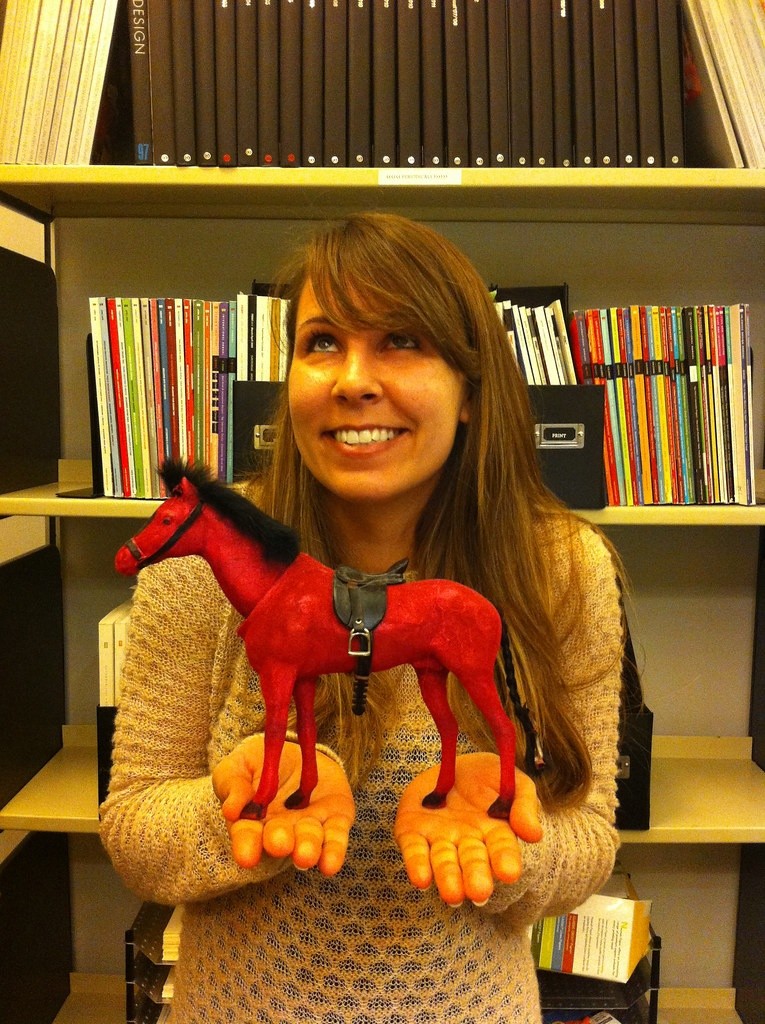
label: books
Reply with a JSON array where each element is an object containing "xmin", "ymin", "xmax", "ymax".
[
  {"xmin": 87, "ymin": 292, "xmax": 756, "ymax": 509},
  {"xmin": 94, "ymin": 597, "xmax": 135, "ymax": 709},
  {"xmin": 0, "ymin": 0, "xmax": 764, "ymax": 167},
  {"xmin": 153, "ymin": 857, "xmax": 653, "ymax": 1024}
]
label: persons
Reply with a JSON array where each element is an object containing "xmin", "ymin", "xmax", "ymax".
[{"xmin": 97, "ymin": 211, "xmax": 647, "ymax": 1024}]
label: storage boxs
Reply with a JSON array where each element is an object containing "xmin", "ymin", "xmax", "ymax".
[
  {"xmin": 230, "ymin": 377, "xmax": 605, "ymax": 512},
  {"xmin": 97, "ymin": 703, "xmax": 119, "ymax": 820}
]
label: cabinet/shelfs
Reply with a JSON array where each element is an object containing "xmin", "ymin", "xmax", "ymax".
[
  {"xmin": 526, "ymin": 863, "xmax": 663, "ymax": 1022},
  {"xmin": 0, "ymin": 162, "xmax": 765, "ymax": 1024},
  {"xmin": 124, "ymin": 894, "xmax": 183, "ymax": 1023}
]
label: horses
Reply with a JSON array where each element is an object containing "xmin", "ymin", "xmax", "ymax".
[{"xmin": 114, "ymin": 456, "xmax": 518, "ymax": 821}]
